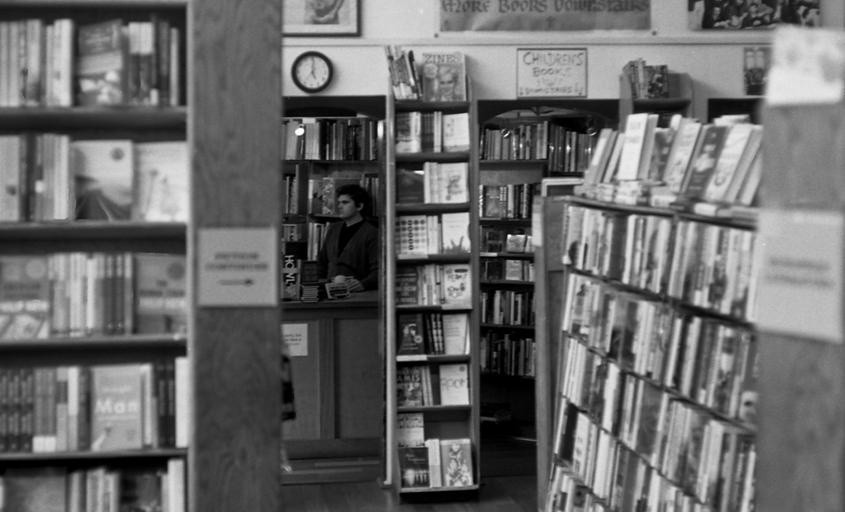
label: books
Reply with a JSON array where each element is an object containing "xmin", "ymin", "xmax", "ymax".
[
  {"xmin": 384, "ymin": 44, "xmax": 770, "ymax": 512},
  {"xmin": 1, "ymin": 13, "xmax": 189, "ymax": 510},
  {"xmin": 282, "ymin": 117, "xmax": 384, "ymax": 218},
  {"xmin": 282, "ymin": 223, "xmax": 329, "ymax": 302}
]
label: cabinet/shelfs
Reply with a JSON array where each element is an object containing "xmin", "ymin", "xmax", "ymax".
[
  {"xmin": 707, "ymin": 96, "xmax": 764, "ymax": 124},
  {"xmin": 384, "ymin": 75, "xmax": 478, "ymax": 499},
  {"xmin": 528, "ymin": 194, "xmax": 765, "ymax": 511},
  {"xmin": 0, "ymin": 0, "xmax": 282, "ymax": 510},
  {"xmin": 282, "ymin": 116, "xmax": 384, "ymax": 288},
  {"xmin": 477, "ymin": 97, "xmax": 692, "ymax": 414}
]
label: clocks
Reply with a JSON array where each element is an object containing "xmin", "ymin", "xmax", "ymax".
[{"xmin": 291, "ymin": 51, "xmax": 332, "ymax": 92}]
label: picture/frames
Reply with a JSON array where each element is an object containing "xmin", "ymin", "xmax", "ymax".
[
  {"xmin": 282, "ymin": 0, "xmax": 361, "ymax": 37},
  {"xmin": 515, "ymin": 47, "xmax": 588, "ymax": 98}
]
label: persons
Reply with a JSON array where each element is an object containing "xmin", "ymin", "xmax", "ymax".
[
  {"xmin": 688, "ymin": 0, "xmax": 823, "ymax": 34},
  {"xmin": 304, "ymin": 1, "xmax": 344, "ymax": 25},
  {"xmin": 316, "ymin": 184, "xmax": 378, "ymax": 294}
]
